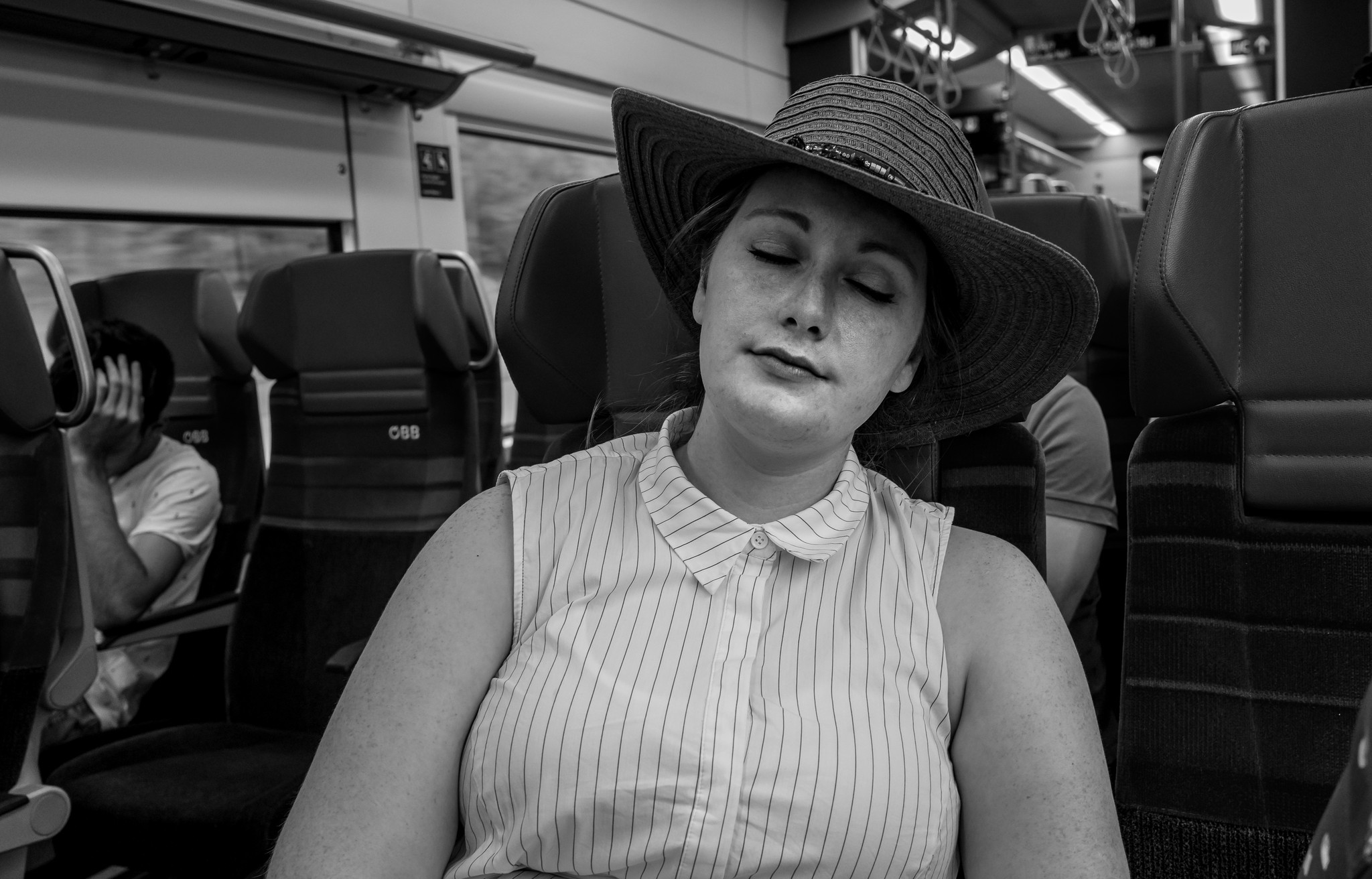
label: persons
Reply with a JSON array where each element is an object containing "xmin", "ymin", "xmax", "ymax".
[
  {"xmin": 35, "ymin": 321, "xmax": 221, "ymax": 767},
  {"xmin": 269, "ymin": 75, "xmax": 1130, "ymax": 879},
  {"xmin": 1017, "ymin": 375, "xmax": 1117, "ymax": 628}
]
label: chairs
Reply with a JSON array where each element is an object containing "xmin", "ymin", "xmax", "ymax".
[{"xmin": 0, "ymin": 85, "xmax": 1371, "ymax": 879}]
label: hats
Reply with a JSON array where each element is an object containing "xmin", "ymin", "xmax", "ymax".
[{"xmin": 608, "ymin": 75, "xmax": 1101, "ymax": 450}]
